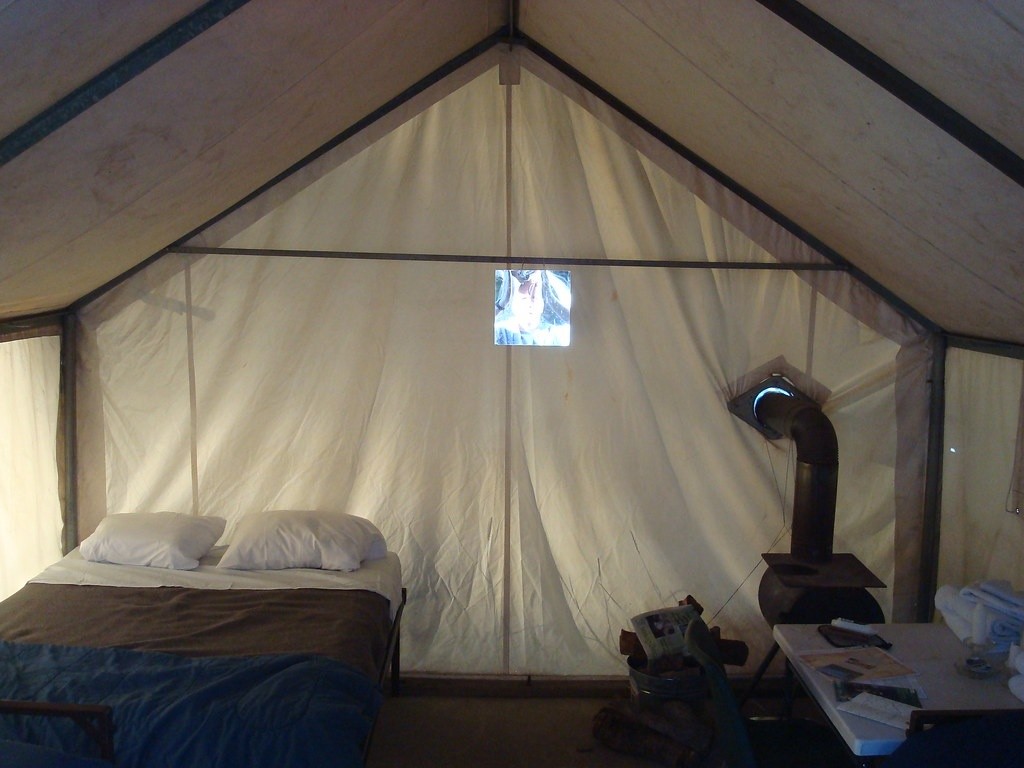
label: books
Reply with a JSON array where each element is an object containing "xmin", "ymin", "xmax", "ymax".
[{"xmin": 803, "ymin": 644, "xmax": 932, "ymax": 733}]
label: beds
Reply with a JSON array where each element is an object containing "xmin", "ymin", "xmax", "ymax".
[{"xmin": 1, "ymin": 544, "xmax": 407, "ymax": 768}]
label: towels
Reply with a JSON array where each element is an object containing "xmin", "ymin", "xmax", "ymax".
[{"xmin": 934, "ymin": 576, "xmax": 1024, "ymax": 655}]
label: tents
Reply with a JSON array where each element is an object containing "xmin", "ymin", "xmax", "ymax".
[{"xmin": 0, "ymin": 0, "xmax": 1024, "ymax": 695}]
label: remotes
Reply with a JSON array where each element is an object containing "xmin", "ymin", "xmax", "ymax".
[{"xmin": 831, "ymin": 620, "xmax": 874, "ymax": 636}]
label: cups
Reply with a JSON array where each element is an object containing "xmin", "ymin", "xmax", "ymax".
[{"xmin": 961, "ymin": 637, "xmax": 995, "ymax": 678}]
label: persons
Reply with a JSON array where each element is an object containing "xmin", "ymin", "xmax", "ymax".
[{"xmin": 495, "ymin": 280, "xmax": 570, "ymax": 346}]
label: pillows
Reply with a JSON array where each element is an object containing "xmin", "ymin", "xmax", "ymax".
[
  {"xmin": 78, "ymin": 511, "xmax": 226, "ymax": 570},
  {"xmin": 218, "ymin": 509, "xmax": 389, "ymax": 572}
]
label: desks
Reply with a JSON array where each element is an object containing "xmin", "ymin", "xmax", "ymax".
[{"xmin": 772, "ymin": 622, "xmax": 1024, "ymax": 757}]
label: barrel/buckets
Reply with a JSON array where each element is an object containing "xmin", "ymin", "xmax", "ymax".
[{"xmin": 627, "ymin": 657, "xmax": 709, "ymax": 718}]
